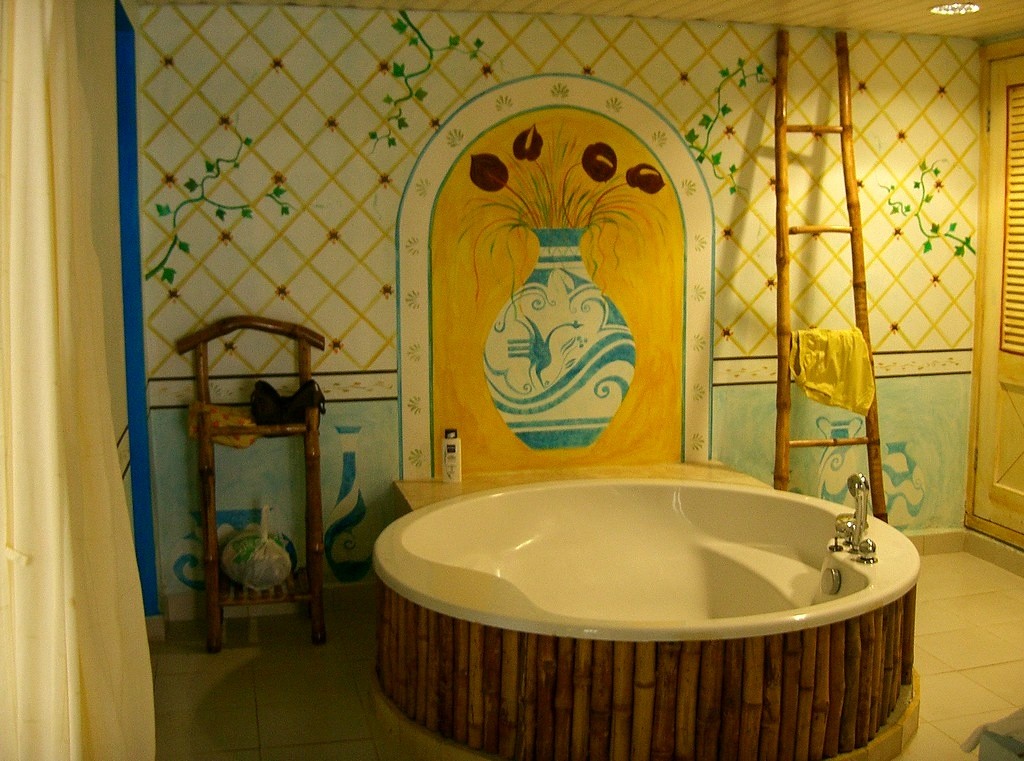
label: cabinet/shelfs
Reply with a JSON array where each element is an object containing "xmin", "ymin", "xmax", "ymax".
[{"xmin": 176, "ymin": 314, "xmax": 327, "ymax": 650}]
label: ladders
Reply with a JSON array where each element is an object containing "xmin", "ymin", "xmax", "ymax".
[{"xmin": 772, "ymin": 29, "xmax": 891, "ymax": 527}]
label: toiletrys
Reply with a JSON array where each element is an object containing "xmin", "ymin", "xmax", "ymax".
[{"xmin": 441, "ymin": 428, "xmax": 462, "ymax": 483}]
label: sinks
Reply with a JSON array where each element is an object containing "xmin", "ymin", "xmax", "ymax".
[{"xmin": 373, "ymin": 478, "xmax": 920, "ymax": 761}]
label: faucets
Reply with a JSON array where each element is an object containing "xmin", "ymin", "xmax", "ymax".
[{"xmin": 847, "ymin": 472, "xmax": 869, "ymax": 554}]
